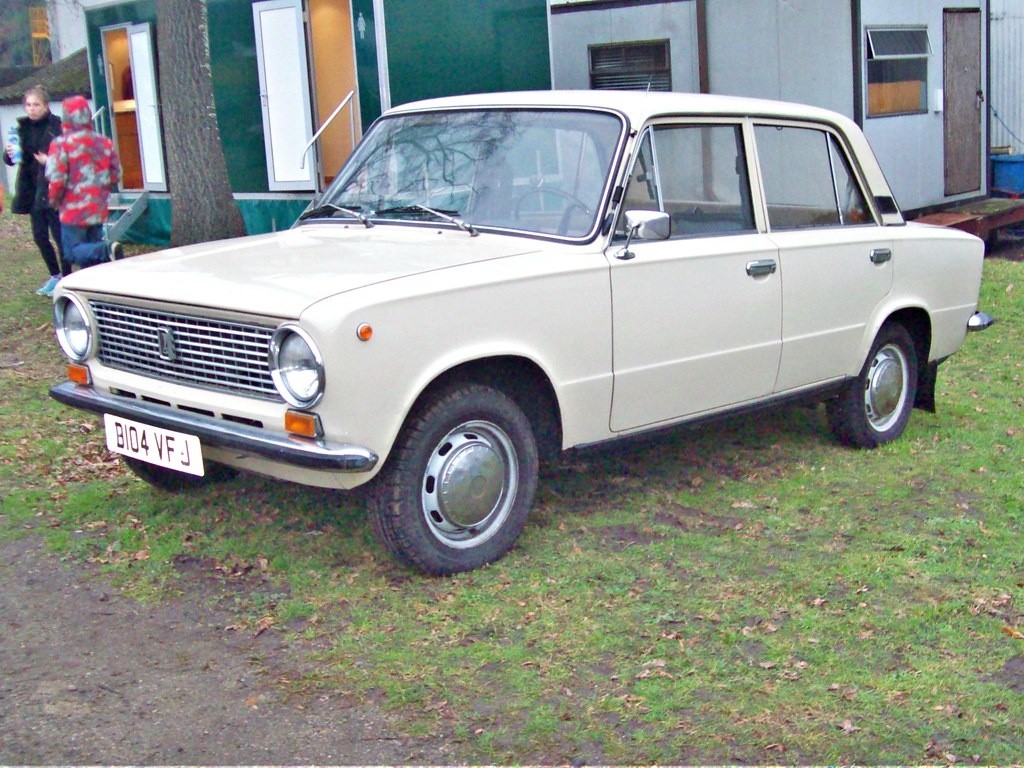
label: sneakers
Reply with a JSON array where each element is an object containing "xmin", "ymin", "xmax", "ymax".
[
  {"xmin": 36, "ymin": 274, "xmax": 61, "ymax": 298},
  {"xmin": 111, "ymin": 242, "xmax": 125, "ymax": 260}
]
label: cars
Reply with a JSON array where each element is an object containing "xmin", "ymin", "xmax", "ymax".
[{"xmin": 47, "ymin": 88, "xmax": 985, "ymax": 578}]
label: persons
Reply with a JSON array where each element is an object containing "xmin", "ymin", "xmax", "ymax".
[
  {"xmin": 45, "ymin": 96, "xmax": 123, "ymax": 269},
  {"xmin": 3, "ymin": 84, "xmax": 73, "ymax": 297}
]
